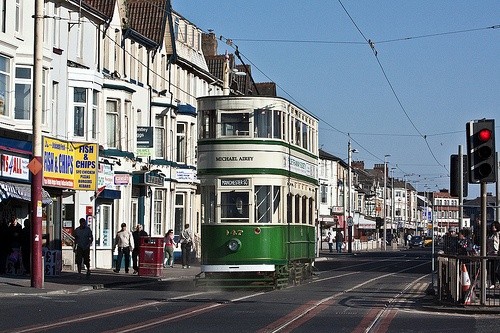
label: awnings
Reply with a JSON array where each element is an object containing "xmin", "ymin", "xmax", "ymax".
[
  {"xmin": 0, "ymin": 180, "xmax": 52, "ymax": 203},
  {"xmin": 98, "ymin": 189, "xmax": 120, "ymax": 199}
]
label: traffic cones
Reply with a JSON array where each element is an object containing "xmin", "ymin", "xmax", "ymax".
[{"xmin": 461, "ymin": 263, "xmax": 475, "ymax": 305}]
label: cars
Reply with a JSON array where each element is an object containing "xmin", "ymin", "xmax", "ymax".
[{"xmin": 409, "ymin": 235, "xmax": 438, "ymax": 249}]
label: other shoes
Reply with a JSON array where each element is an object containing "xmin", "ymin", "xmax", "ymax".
[
  {"xmin": 125, "ymin": 269, "xmax": 129, "ymax": 273},
  {"xmin": 182, "ymin": 266, "xmax": 190, "ymax": 269},
  {"xmin": 133, "ymin": 270, "xmax": 138, "ymax": 274},
  {"xmin": 169, "ymin": 266, "xmax": 173, "ymax": 268},
  {"xmin": 113, "ymin": 269, "xmax": 119, "ymax": 273},
  {"xmin": 163, "ymin": 264, "xmax": 166, "ymax": 268},
  {"xmin": 86, "ymin": 270, "xmax": 91, "ymax": 277},
  {"xmin": 490, "ymin": 285, "xmax": 495, "ymax": 289},
  {"xmin": 76, "ymin": 274, "xmax": 83, "ymax": 279}
]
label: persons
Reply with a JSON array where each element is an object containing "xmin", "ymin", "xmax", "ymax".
[
  {"xmin": 176, "ymin": 224, "xmax": 194, "ymax": 269},
  {"xmin": 328, "ymin": 232, "xmax": 335, "ymax": 252},
  {"xmin": 73, "ymin": 218, "xmax": 93, "ymax": 280},
  {"xmin": 444, "ymin": 226, "xmax": 472, "ymax": 281},
  {"xmin": 112, "ymin": 223, "xmax": 135, "ymax": 273},
  {"xmin": 486, "ymin": 221, "xmax": 500, "ymax": 289},
  {"xmin": 0, "ymin": 219, "xmax": 30, "ymax": 278},
  {"xmin": 404, "ymin": 233, "xmax": 412, "ymax": 246},
  {"xmin": 163, "ymin": 229, "xmax": 178, "ymax": 268},
  {"xmin": 335, "ymin": 231, "xmax": 343, "ymax": 254},
  {"xmin": 132, "ymin": 224, "xmax": 149, "ymax": 274}
]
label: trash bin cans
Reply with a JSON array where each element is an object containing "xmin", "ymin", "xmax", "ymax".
[
  {"xmin": 138, "ymin": 237, "xmax": 165, "ymax": 276},
  {"xmin": 393, "ymin": 241, "xmax": 397, "ymax": 249},
  {"xmin": 381, "ymin": 241, "xmax": 384, "ymax": 250}
]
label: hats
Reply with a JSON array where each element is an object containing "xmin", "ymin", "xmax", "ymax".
[
  {"xmin": 121, "ymin": 223, "xmax": 126, "ymax": 227},
  {"xmin": 448, "ymin": 227, "xmax": 455, "ymax": 232}
]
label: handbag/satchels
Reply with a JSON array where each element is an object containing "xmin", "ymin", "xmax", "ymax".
[{"xmin": 467, "ymin": 247, "xmax": 478, "ymax": 262}]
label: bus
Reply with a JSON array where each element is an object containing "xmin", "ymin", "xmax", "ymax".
[{"xmin": 195, "ymin": 51, "xmax": 322, "ymax": 291}]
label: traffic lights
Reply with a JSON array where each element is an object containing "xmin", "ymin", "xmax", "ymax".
[{"xmin": 466, "ymin": 118, "xmax": 497, "ymax": 183}]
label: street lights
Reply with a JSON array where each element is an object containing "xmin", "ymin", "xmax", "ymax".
[
  {"xmin": 390, "ymin": 167, "xmax": 397, "ymax": 250},
  {"xmin": 347, "ymin": 139, "xmax": 359, "ymax": 254},
  {"xmin": 404, "ymin": 181, "xmax": 410, "ymax": 229},
  {"xmin": 383, "ymin": 154, "xmax": 391, "ymax": 250},
  {"xmin": 422, "ymin": 186, "xmax": 431, "ymax": 236}
]
